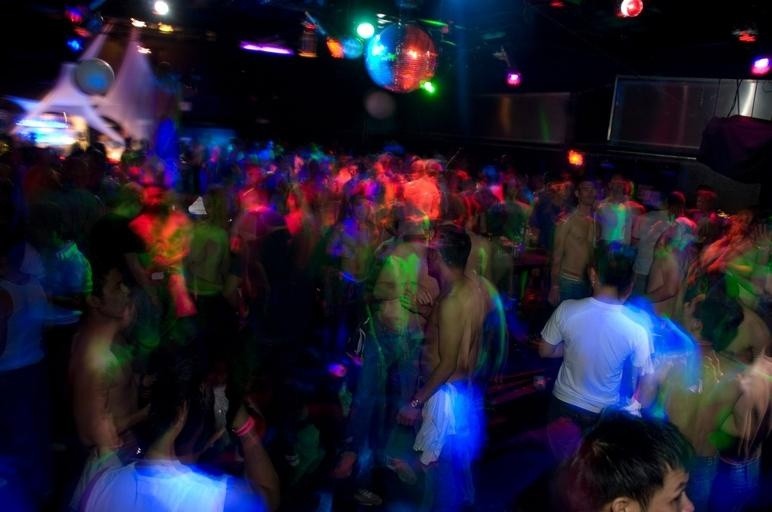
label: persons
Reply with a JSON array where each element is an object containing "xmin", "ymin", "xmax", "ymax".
[{"xmin": 1, "ymin": 126, "xmax": 772, "ymax": 512}]
